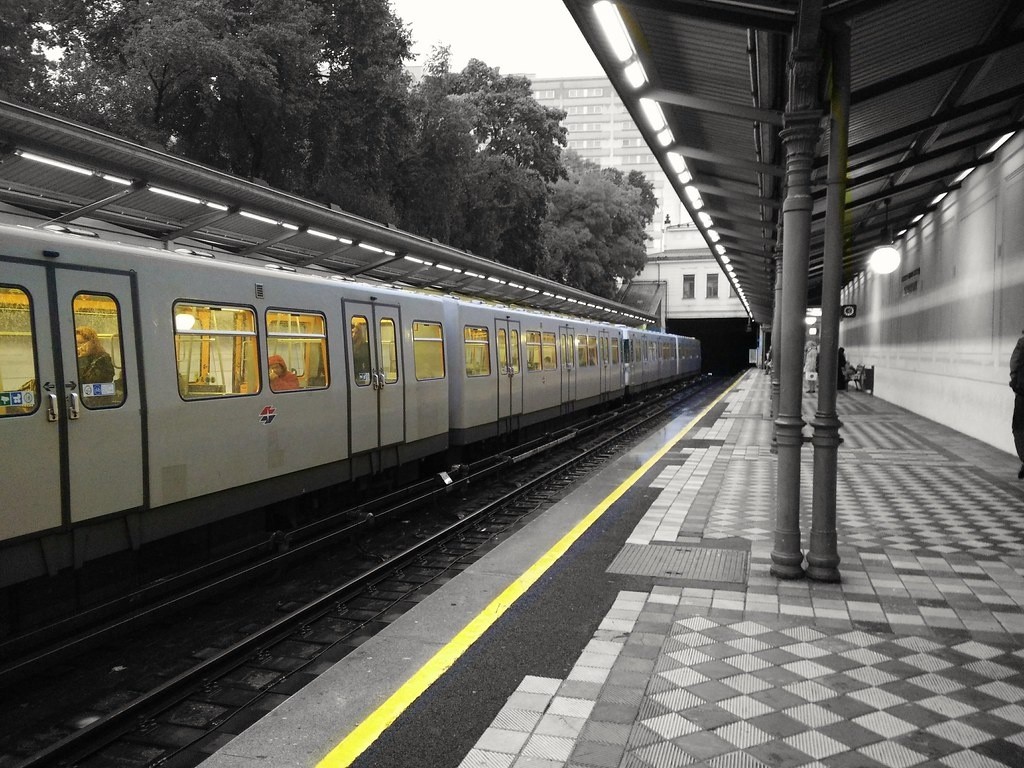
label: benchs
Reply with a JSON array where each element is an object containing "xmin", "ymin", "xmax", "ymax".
[
  {"xmin": 845, "ymin": 364, "xmax": 865, "ymax": 392},
  {"xmin": 184, "ymin": 384, "xmax": 224, "ymax": 397}
]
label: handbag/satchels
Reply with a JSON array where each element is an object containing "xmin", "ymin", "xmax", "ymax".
[{"xmin": 806, "ymin": 372, "xmax": 818, "ymax": 380}]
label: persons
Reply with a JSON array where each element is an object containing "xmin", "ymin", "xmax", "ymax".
[
  {"xmin": 765, "ymin": 346, "xmax": 773, "ymax": 375},
  {"xmin": 351, "ymin": 323, "xmax": 370, "ymax": 386},
  {"xmin": 1008, "ymin": 328, "xmax": 1024, "ymax": 479},
  {"xmin": 804, "ymin": 341, "xmax": 865, "ymax": 393},
  {"xmin": 269, "ymin": 355, "xmax": 300, "ymax": 392},
  {"xmin": 75, "ymin": 326, "xmax": 114, "ymax": 407}
]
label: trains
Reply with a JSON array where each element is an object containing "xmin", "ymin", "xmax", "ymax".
[{"xmin": 1, "ymin": 222, "xmax": 702, "ymax": 591}]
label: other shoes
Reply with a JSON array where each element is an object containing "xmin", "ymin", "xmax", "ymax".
[
  {"xmin": 806, "ymin": 390, "xmax": 811, "ymax": 393},
  {"xmin": 763, "ymin": 373, "xmax": 768, "ymax": 375},
  {"xmin": 810, "ymin": 391, "xmax": 815, "ymax": 393}
]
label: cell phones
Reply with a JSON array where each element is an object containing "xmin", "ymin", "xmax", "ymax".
[{"xmin": 78, "ymin": 340, "xmax": 94, "ymax": 354}]
